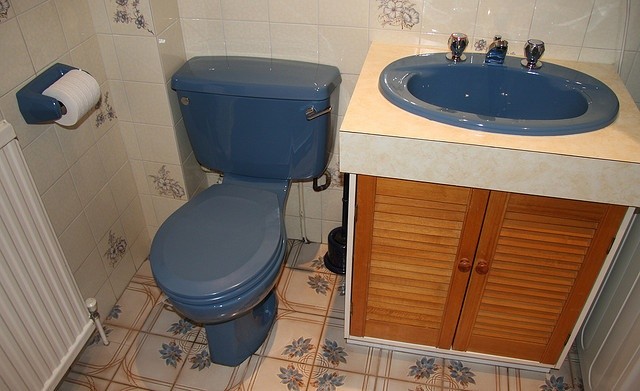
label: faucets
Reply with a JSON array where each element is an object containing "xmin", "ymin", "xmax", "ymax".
[{"xmin": 485, "ymin": 40, "xmax": 508, "ymax": 62}]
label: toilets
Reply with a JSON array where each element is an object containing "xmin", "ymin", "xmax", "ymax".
[{"xmin": 150, "ymin": 57, "xmax": 342, "ymax": 366}]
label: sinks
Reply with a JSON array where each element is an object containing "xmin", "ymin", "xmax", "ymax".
[{"xmin": 408, "ymin": 64, "xmax": 589, "ymax": 120}]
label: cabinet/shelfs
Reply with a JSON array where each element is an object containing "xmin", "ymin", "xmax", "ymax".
[{"xmin": 349, "ymin": 174, "xmax": 630, "ymax": 368}]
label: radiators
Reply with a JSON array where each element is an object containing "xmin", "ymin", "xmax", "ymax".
[{"xmin": 0, "ymin": 120, "xmax": 110, "ymax": 390}]
